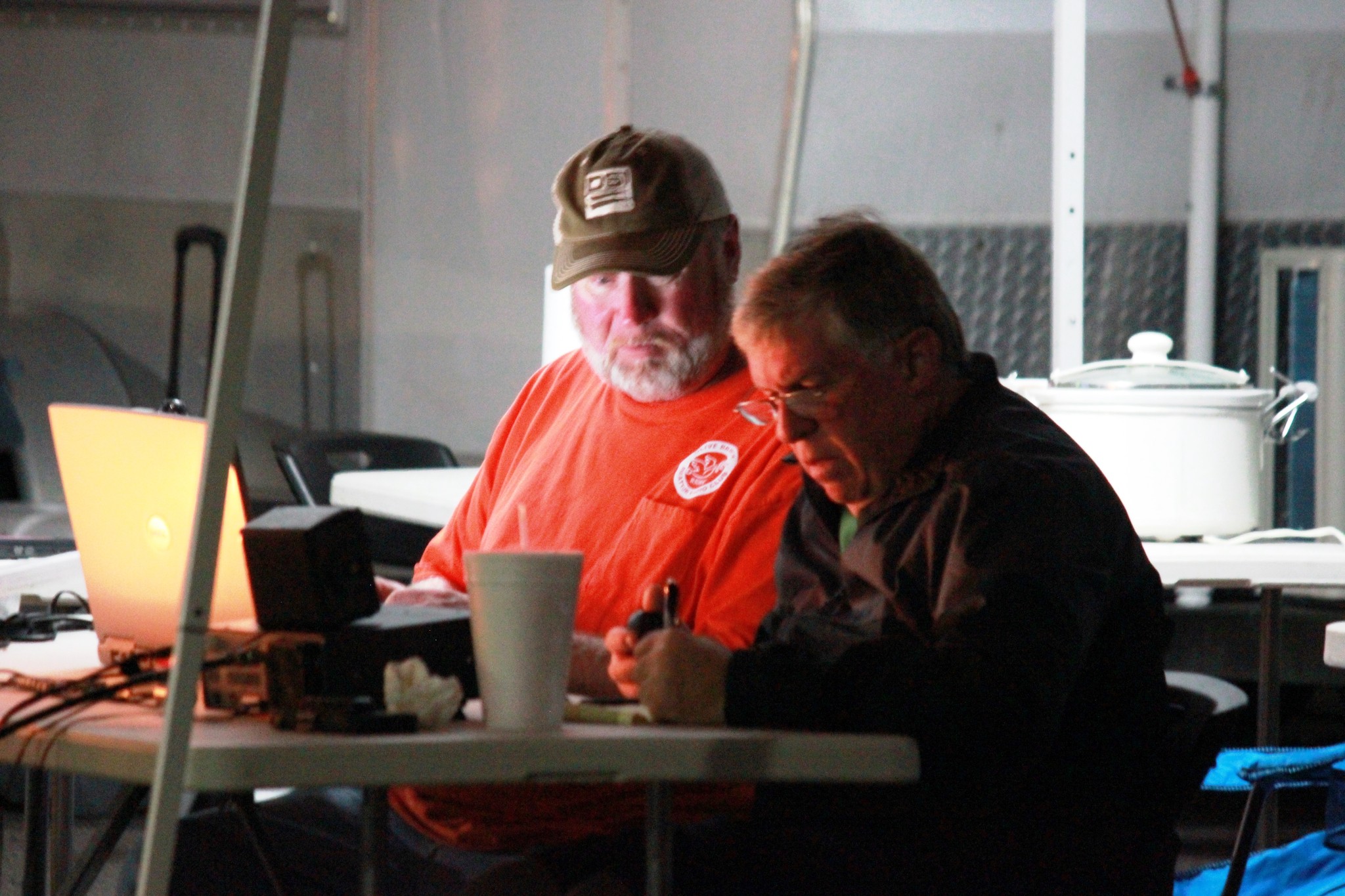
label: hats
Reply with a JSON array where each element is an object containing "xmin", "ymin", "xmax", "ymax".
[{"xmin": 550, "ymin": 127, "xmax": 730, "ymax": 292}]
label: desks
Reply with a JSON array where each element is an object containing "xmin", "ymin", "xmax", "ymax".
[
  {"xmin": 1142, "ymin": 543, "xmax": 1345, "ymax": 841},
  {"xmin": 0, "ymin": 680, "xmax": 918, "ymax": 896}
]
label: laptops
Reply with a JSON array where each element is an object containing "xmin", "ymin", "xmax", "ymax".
[{"xmin": 46, "ymin": 404, "xmax": 262, "ymax": 662}]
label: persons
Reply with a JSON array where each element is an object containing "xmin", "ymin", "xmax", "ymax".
[
  {"xmin": 606, "ymin": 213, "xmax": 1188, "ymax": 896},
  {"xmin": 116, "ymin": 123, "xmax": 807, "ymax": 896}
]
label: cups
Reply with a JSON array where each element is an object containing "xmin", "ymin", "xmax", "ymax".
[{"xmin": 461, "ymin": 548, "xmax": 584, "ymax": 733}]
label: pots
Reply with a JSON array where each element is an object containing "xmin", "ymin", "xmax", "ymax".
[{"xmin": 1037, "ymin": 331, "xmax": 1276, "ymax": 540}]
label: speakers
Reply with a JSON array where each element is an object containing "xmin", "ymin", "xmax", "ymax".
[{"xmin": 242, "ymin": 507, "xmax": 379, "ymax": 632}]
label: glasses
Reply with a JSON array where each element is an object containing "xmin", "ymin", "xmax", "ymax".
[{"xmin": 734, "ymin": 329, "xmax": 921, "ymax": 428}]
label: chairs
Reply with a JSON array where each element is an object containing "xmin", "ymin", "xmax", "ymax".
[{"xmin": 271, "ymin": 432, "xmax": 457, "ymax": 507}]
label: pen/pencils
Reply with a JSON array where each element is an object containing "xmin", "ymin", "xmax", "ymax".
[{"xmin": 662, "ymin": 577, "xmax": 679, "ymax": 627}]
